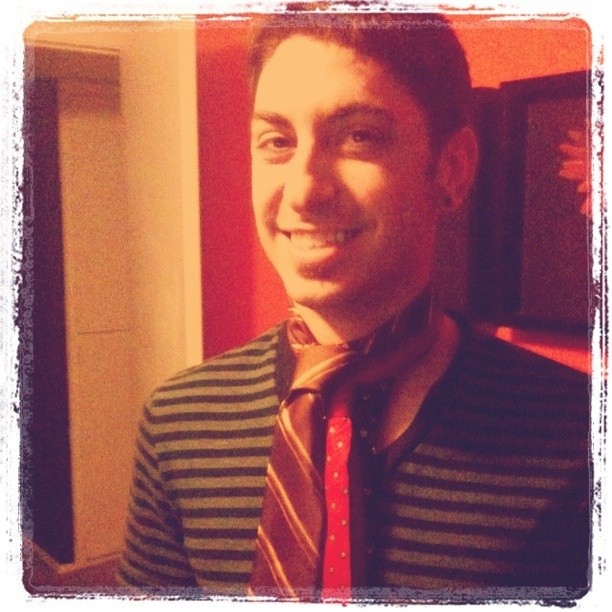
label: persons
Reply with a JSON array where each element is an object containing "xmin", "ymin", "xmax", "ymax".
[{"xmin": 113, "ymin": 13, "xmax": 591, "ymax": 587}]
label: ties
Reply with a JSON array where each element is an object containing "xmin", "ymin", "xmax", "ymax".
[{"xmin": 247, "ymin": 282, "xmax": 443, "ymax": 601}]
